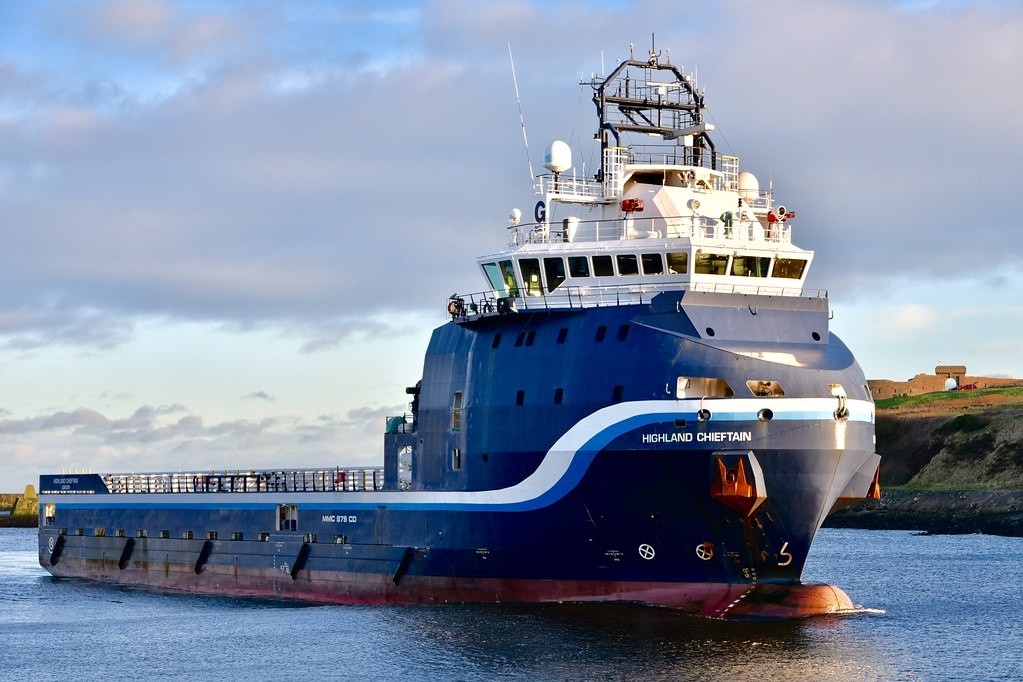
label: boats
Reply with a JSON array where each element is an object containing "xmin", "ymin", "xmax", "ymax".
[{"xmin": 39, "ymin": 36, "xmax": 880, "ymax": 622}]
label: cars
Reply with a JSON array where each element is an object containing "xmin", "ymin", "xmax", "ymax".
[{"xmin": 952, "ymin": 383, "xmax": 977, "ymax": 390}]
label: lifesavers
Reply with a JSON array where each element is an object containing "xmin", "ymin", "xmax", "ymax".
[{"xmin": 193, "ymin": 476, "xmax": 199, "ymax": 488}]
